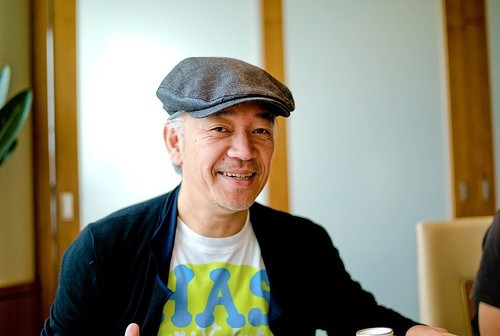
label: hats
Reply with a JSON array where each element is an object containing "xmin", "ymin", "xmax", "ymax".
[{"xmin": 156, "ymin": 57, "xmax": 295, "ymax": 119}]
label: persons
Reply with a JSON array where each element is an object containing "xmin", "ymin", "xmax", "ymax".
[
  {"xmin": 41, "ymin": 58, "xmax": 459, "ymax": 336},
  {"xmin": 469, "ymin": 207, "xmax": 500, "ymax": 336}
]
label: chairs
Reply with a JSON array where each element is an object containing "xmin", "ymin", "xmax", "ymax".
[{"xmin": 416, "ymin": 215, "xmax": 494, "ymax": 336}]
